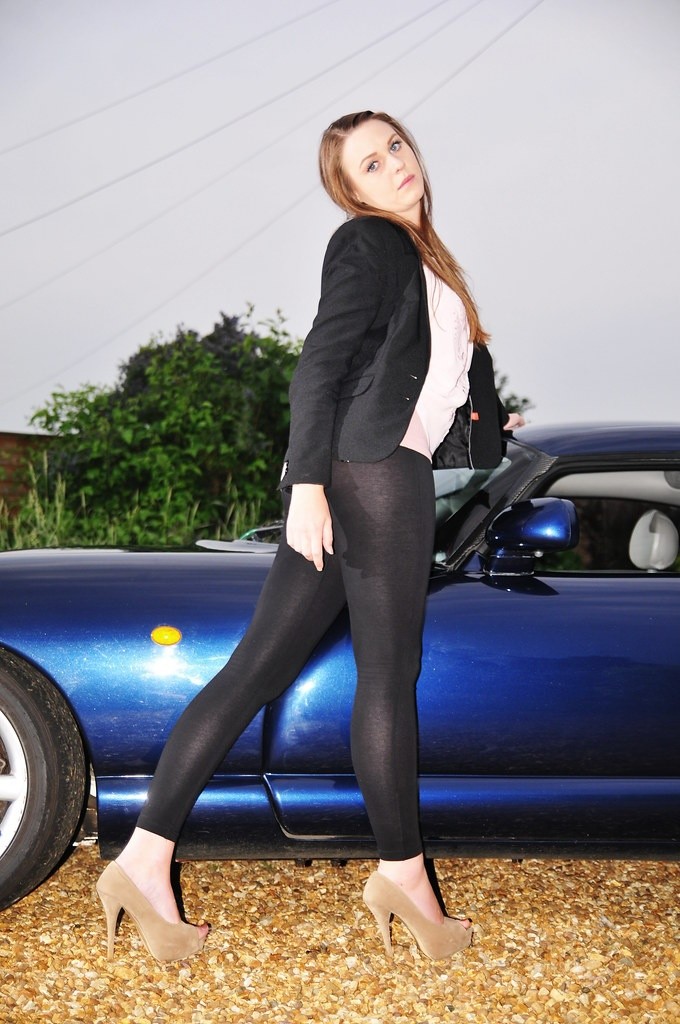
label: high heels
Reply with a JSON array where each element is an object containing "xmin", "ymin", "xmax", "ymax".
[
  {"xmin": 94, "ymin": 861, "xmax": 205, "ymax": 963},
  {"xmin": 363, "ymin": 872, "xmax": 473, "ymax": 959}
]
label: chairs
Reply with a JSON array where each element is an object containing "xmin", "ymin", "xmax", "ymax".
[{"xmin": 629, "ymin": 509, "xmax": 680, "ymax": 573}]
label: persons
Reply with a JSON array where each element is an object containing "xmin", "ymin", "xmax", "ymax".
[{"xmin": 99, "ymin": 110, "xmax": 531, "ymax": 961}]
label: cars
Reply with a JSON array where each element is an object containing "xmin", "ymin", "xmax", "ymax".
[{"xmin": 0, "ymin": 418, "xmax": 678, "ymax": 911}]
